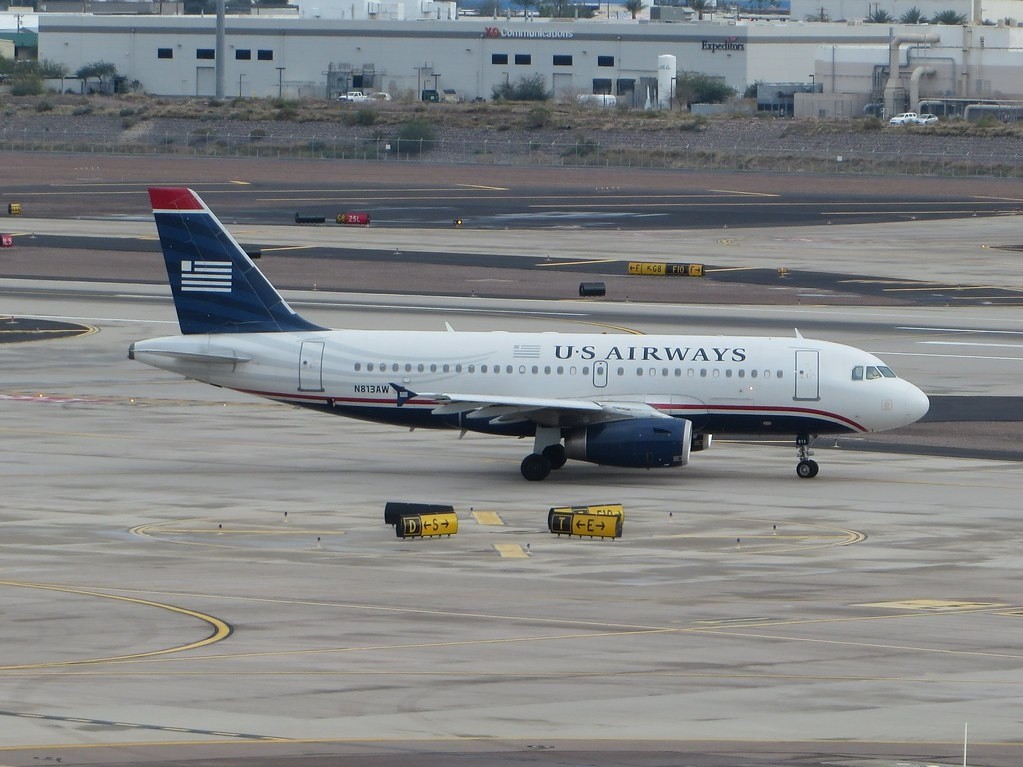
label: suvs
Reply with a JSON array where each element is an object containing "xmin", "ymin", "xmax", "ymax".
[{"xmin": 890, "ymin": 112, "xmax": 917, "ymax": 126}]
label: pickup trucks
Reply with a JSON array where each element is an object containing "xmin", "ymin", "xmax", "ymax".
[{"xmin": 336, "ymin": 91, "xmax": 367, "ymax": 103}]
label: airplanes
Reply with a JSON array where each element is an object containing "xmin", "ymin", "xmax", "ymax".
[{"xmin": 124, "ymin": 186, "xmax": 932, "ymax": 484}]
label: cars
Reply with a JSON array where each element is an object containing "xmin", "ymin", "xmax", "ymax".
[
  {"xmin": 367, "ymin": 92, "xmax": 393, "ymax": 104},
  {"xmin": 914, "ymin": 113, "xmax": 939, "ymax": 125}
]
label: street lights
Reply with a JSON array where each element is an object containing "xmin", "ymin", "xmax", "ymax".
[
  {"xmin": 670, "ymin": 77, "xmax": 677, "ymax": 111},
  {"xmin": 502, "ymin": 72, "xmax": 509, "ymax": 97},
  {"xmin": 430, "ymin": 73, "xmax": 442, "ymax": 90},
  {"xmin": 276, "ymin": 67, "xmax": 286, "ymax": 98},
  {"xmin": 239, "ymin": 73, "xmax": 247, "ymax": 97},
  {"xmin": 809, "ymin": 74, "xmax": 815, "ymax": 92}
]
